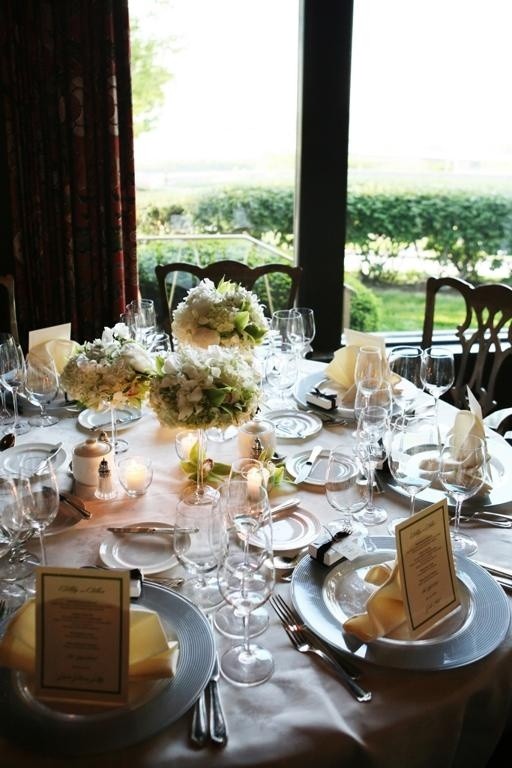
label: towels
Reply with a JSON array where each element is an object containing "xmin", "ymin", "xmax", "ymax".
[
  {"xmin": 1, "ymin": 597, "xmax": 180, "ymax": 681},
  {"xmin": 422, "ymin": 380, "xmax": 492, "ymax": 498},
  {"xmin": 13, "ymin": 335, "xmax": 81, "ymax": 388},
  {"xmin": 324, "ymin": 328, "xmax": 403, "ymax": 404},
  {"xmin": 342, "ymin": 554, "xmax": 456, "ymax": 643}
]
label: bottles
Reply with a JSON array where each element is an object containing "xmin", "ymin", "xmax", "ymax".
[{"xmin": 94, "ymin": 457, "xmax": 115, "ymax": 501}]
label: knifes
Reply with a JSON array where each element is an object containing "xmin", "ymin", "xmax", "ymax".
[
  {"xmin": 43, "ymin": 484, "xmax": 93, "ymax": 521},
  {"xmin": 293, "ymin": 444, "xmax": 326, "ymax": 487},
  {"xmin": 89, "ymin": 414, "xmax": 141, "ymax": 432},
  {"xmin": 105, "ymin": 524, "xmax": 201, "ymax": 537},
  {"xmin": 190, "ymin": 648, "xmax": 232, "ymax": 751}
]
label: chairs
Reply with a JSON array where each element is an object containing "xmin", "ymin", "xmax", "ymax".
[
  {"xmin": 415, "ymin": 276, "xmax": 512, "ymax": 447},
  {"xmin": 155, "ymin": 261, "xmax": 304, "ymax": 352}
]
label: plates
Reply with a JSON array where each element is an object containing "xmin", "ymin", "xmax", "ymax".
[
  {"xmin": 2, "ymin": 490, "xmax": 87, "ymax": 538},
  {"xmin": 1, "ymin": 442, "xmax": 68, "ymax": 474},
  {"xmin": 1, "ymin": 581, "xmax": 215, "ymax": 754},
  {"xmin": 286, "ymin": 449, "xmax": 358, "ymax": 487},
  {"xmin": 288, "ymin": 532, "xmax": 512, "ymax": 673},
  {"xmin": 296, "ymin": 368, "xmax": 368, "ymax": 416},
  {"xmin": 22, "ymin": 394, "xmax": 78, "ymax": 409},
  {"xmin": 391, "ymin": 419, "xmax": 511, "ymax": 509},
  {"xmin": 255, "ymin": 525, "xmax": 334, "ymax": 570},
  {"xmin": 94, "ymin": 520, "xmax": 193, "ymax": 577},
  {"xmin": 263, "ymin": 407, "xmax": 325, "ymax": 440},
  {"xmin": 238, "ymin": 503, "xmax": 323, "ymax": 552},
  {"xmin": 76, "ymin": 401, "xmax": 143, "ymax": 431}
]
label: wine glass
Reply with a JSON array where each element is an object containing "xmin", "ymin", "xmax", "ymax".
[
  {"xmin": 387, "ymin": 412, "xmax": 441, "ymax": 534},
  {"xmin": 350, "ymin": 344, "xmax": 392, "ymax": 526},
  {"xmin": 388, "ymin": 344, "xmax": 459, "ymax": 419},
  {"xmin": 119, "ymin": 298, "xmax": 172, "ymax": 354},
  {"xmin": 172, "ymin": 494, "xmax": 223, "ymax": 612},
  {"xmin": 0, "ymin": 449, "xmax": 58, "ymax": 622},
  {"xmin": 438, "ymin": 433, "xmax": 491, "ymax": 558},
  {"xmin": 206, "ymin": 459, "xmax": 277, "ymax": 691},
  {"xmin": 0, "ymin": 333, "xmax": 62, "ymax": 436},
  {"xmin": 327, "ymin": 444, "xmax": 374, "ymax": 539},
  {"xmin": 264, "ymin": 303, "xmax": 317, "ymax": 404}
]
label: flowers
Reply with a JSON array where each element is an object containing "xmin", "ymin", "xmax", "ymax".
[
  {"xmin": 149, "ymin": 347, "xmax": 263, "ymax": 431},
  {"xmin": 169, "ymin": 277, "xmax": 270, "ymax": 354},
  {"xmin": 57, "ymin": 330, "xmax": 159, "ymax": 411}
]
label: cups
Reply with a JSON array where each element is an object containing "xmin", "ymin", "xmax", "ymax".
[
  {"xmin": 115, "ymin": 456, "xmax": 155, "ymax": 497},
  {"xmin": 174, "ymin": 426, "xmax": 211, "ymax": 464},
  {"xmin": 67, "ymin": 438, "xmax": 116, "ymax": 486},
  {"xmin": 237, "ymin": 416, "xmax": 280, "ymax": 460}
]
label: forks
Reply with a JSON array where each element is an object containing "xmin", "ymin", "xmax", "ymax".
[
  {"xmin": 33, "ymin": 442, "xmax": 63, "ymax": 471},
  {"xmin": 270, "ymin": 592, "xmax": 374, "ymax": 703}
]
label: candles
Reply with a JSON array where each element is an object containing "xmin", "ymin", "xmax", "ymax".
[
  {"xmin": 126, "ymin": 464, "xmax": 149, "ymax": 491},
  {"xmin": 245, "ymin": 468, "xmax": 265, "ymax": 502},
  {"xmin": 181, "ymin": 435, "xmax": 198, "ymax": 459},
  {"xmin": 237, "ymin": 419, "xmax": 275, "ymax": 458}
]
